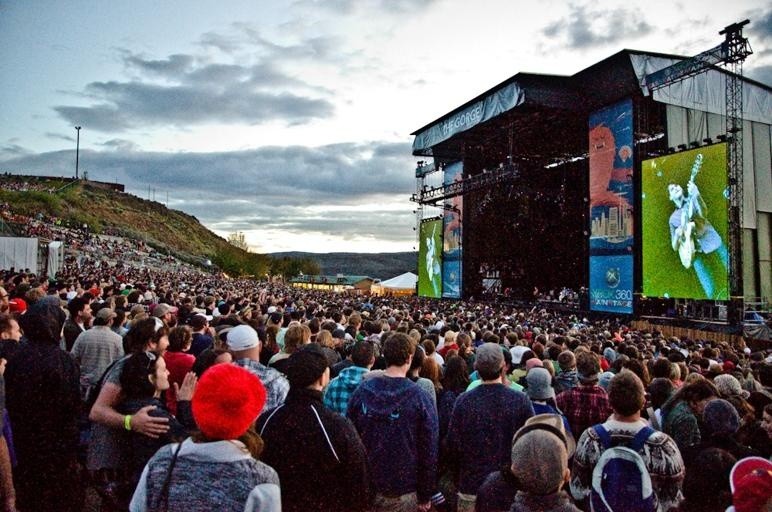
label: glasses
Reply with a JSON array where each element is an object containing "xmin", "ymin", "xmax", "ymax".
[
  {"xmin": 151, "ymin": 316, "xmax": 164, "ymax": 331},
  {"xmin": 145, "ymin": 351, "xmax": 156, "ymax": 368}
]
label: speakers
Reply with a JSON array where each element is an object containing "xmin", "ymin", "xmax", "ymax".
[{"xmin": 727, "ymin": 299, "xmax": 743, "ymax": 321}]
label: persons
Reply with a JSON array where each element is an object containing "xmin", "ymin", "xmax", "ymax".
[
  {"xmin": 665, "ymin": 178, "xmax": 730, "ymax": 299},
  {"xmin": 1, "ymin": 201, "xmax": 772, "ymax": 512},
  {"xmin": 1, "ymin": 181, "xmax": 45, "ymax": 192}
]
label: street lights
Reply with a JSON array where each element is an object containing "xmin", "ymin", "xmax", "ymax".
[{"xmin": 75, "ymin": 126, "xmax": 82, "ymax": 181}]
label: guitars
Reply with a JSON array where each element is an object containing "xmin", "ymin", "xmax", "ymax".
[
  {"xmin": 427, "ymin": 225, "xmax": 437, "ymax": 282},
  {"xmin": 676, "ymin": 154, "xmax": 703, "ymax": 269}
]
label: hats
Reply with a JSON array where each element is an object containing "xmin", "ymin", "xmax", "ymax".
[
  {"xmin": 267, "ymin": 306, "xmax": 285, "ymax": 324},
  {"xmin": 474, "ymin": 342, "xmax": 615, "ymax": 399},
  {"xmin": 192, "ymin": 362, "xmax": 267, "ymax": 439},
  {"xmin": 287, "ymin": 342, "xmax": 337, "ymax": 379},
  {"xmin": 154, "ymin": 303, "xmax": 178, "ymax": 318},
  {"xmin": 226, "ymin": 324, "xmax": 259, "ymax": 351},
  {"xmin": 511, "ymin": 413, "xmax": 575, "ymax": 492},
  {"xmin": 715, "ymin": 374, "xmax": 750, "ymax": 398},
  {"xmin": 444, "ymin": 330, "xmax": 455, "ymax": 345},
  {"xmin": 10, "ymin": 298, "xmax": 26, "ymax": 313},
  {"xmin": 704, "ymin": 399, "xmax": 739, "ymax": 435},
  {"xmin": 191, "ymin": 313, "xmax": 213, "ymax": 326},
  {"xmin": 97, "ymin": 307, "xmax": 117, "ymax": 324},
  {"xmin": 729, "ymin": 455, "xmax": 771, "ymax": 512}
]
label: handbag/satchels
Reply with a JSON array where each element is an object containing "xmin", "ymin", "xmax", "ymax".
[{"xmin": 102, "ymin": 477, "xmax": 135, "ymax": 511}]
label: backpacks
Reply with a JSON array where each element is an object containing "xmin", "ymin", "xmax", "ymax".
[{"xmin": 590, "ymin": 423, "xmax": 661, "ymax": 512}]
